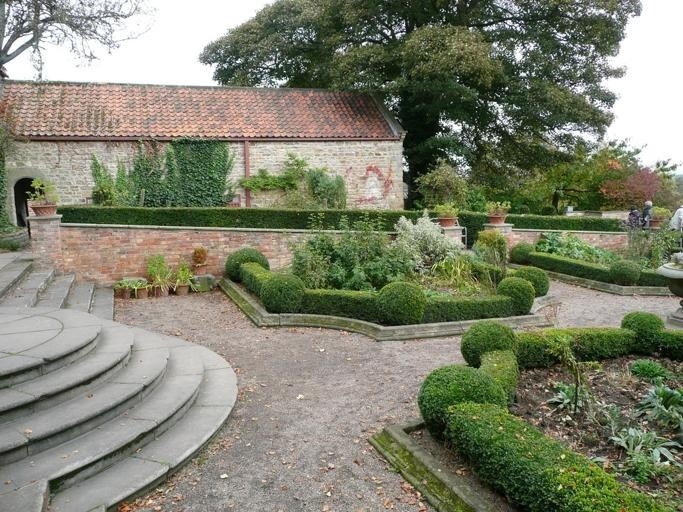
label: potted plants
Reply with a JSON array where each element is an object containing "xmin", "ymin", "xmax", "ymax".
[
  {"xmin": 134, "ymin": 279, "xmax": 149, "ymax": 299},
  {"xmin": 484, "ymin": 200, "xmax": 511, "ymax": 224},
  {"xmin": 146, "ymin": 255, "xmax": 174, "ymax": 297},
  {"xmin": 173, "ymin": 258, "xmax": 198, "ymax": 296},
  {"xmin": 113, "ymin": 279, "xmax": 134, "ymax": 299},
  {"xmin": 24, "ymin": 175, "xmax": 60, "ymax": 216},
  {"xmin": 190, "ymin": 246, "xmax": 209, "ymax": 276},
  {"xmin": 431, "ymin": 202, "xmax": 460, "ymax": 227},
  {"xmin": 648, "ymin": 206, "xmax": 671, "ymax": 228}
]
